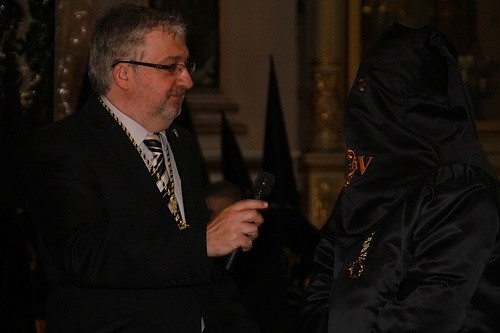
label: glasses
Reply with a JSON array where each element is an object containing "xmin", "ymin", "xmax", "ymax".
[{"xmin": 111, "ymin": 58, "xmax": 196, "ymax": 76}]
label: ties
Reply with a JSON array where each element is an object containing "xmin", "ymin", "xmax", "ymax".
[{"xmin": 144, "ymin": 131, "xmax": 185, "ymax": 229}]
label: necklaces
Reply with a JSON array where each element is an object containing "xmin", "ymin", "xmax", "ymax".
[{"xmin": 96, "ymin": 97, "xmax": 190, "ymax": 233}]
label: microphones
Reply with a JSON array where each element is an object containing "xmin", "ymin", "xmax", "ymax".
[{"xmin": 224, "ymin": 167, "xmax": 279, "ymax": 269}]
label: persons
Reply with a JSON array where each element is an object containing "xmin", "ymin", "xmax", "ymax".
[
  {"xmin": 214, "ymin": 151, "xmax": 330, "ymax": 333},
  {"xmin": 303, "ymin": 24, "xmax": 500, "ymax": 332},
  {"xmin": 2, "ymin": 4, "xmax": 269, "ymax": 333}
]
